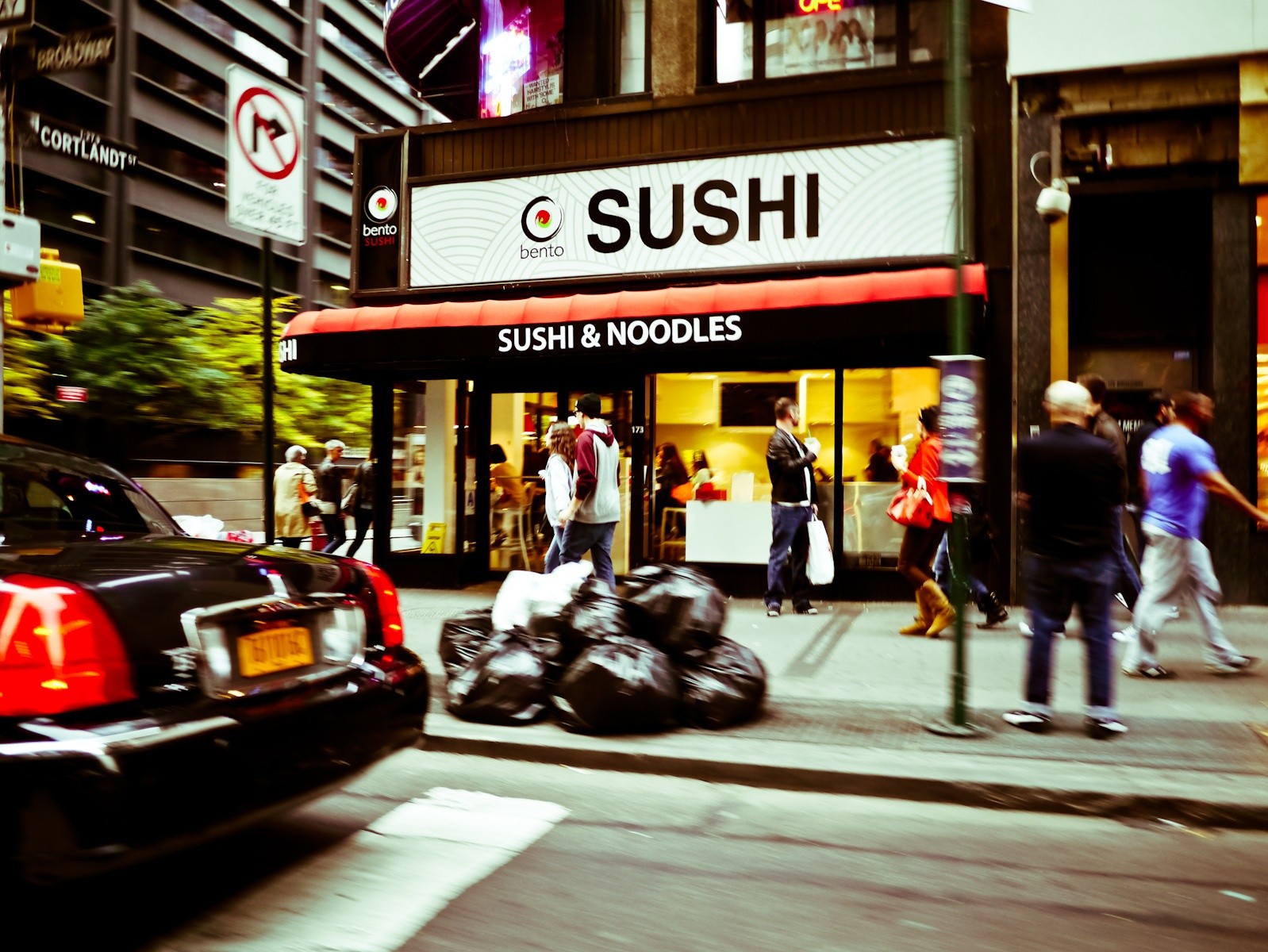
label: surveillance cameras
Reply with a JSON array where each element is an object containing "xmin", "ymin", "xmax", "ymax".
[{"xmin": 1034, "ymin": 177, "xmax": 1071, "ymax": 224}]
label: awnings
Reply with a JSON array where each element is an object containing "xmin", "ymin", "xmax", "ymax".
[{"xmin": 279, "ymin": 263, "xmax": 989, "ymax": 382}]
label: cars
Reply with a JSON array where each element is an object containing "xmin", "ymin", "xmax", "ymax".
[{"xmin": 0, "ymin": 434, "xmax": 428, "ymax": 868}]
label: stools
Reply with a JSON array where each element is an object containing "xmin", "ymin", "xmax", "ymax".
[{"xmin": 660, "ymin": 507, "xmax": 687, "ymax": 558}]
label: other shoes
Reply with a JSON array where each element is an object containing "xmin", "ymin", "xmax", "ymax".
[
  {"xmin": 1111, "ymin": 625, "xmax": 1156, "ymax": 643},
  {"xmin": 1165, "ymin": 606, "xmax": 1179, "ymax": 620},
  {"xmin": 792, "ymin": 603, "xmax": 817, "ymax": 614},
  {"xmin": 1002, "ymin": 707, "xmax": 1052, "ymax": 735},
  {"xmin": 1083, "ymin": 714, "xmax": 1126, "ymax": 740},
  {"xmin": 491, "ymin": 531, "xmax": 508, "ymax": 548},
  {"xmin": 1211, "ymin": 655, "xmax": 1259, "ymax": 675},
  {"xmin": 1122, "ymin": 661, "xmax": 1175, "ymax": 681},
  {"xmin": 1019, "ymin": 622, "xmax": 1066, "ymax": 639},
  {"xmin": 767, "ymin": 605, "xmax": 780, "ymax": 616}
]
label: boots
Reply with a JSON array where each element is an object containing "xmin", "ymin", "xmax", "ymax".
[
  {"xmin": 975, "ymin": 592, "xmax": 1009, "ymax": 628},
  {"xmin": 900, "ymin": 588, "xmax": 935, "ymax": 636},
  {"xmin": 917, "ymin": 579, "xmax": 957, "ymax": 636}
]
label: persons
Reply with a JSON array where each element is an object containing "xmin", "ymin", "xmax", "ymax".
[
  {"xmin": 538, "ymin": 422, "xmax": 577, "ymax": 574},
  {"xmin": 273, "ymin": 445, "xmax": 318, "ymax": 548},
  {"xmin": 347, "ymin": 443, "xmax": 373, "ymax": 557},
  {"xmin": 785, "ymin": 18, "xmax": 871, "ymax": 74},
  {"xmin": 862, "ymin": 437, "xmax": 899, "ymax": 482},
  {"xmin": 1015, "ymin": 379, "xmax": 1128, "ymax": 741},
  {"xmin": 490, "ymin": 444, "xmax": 528, "ymax": 547},
  {"xmin": 891, "ymin": 404, "xmax": 1009, "ymax": 638},
  {"xmin": 1122, "ymin": 389, "xmax": 1268, "ymax": 678},
  {"xmin": 650, "ymin": 442, "xmax": 690, "ymax": 544},
  {"xmin": 558, "ymin": 393, "xmax": 621, "ymax": 593},
  {"xmin": 690, "ymin": 450, "xmax": 714, "ymax": 485},
  {"xmin": 1018, "ymin": 373, "xmax": 1143, "ymax": 641},
  {"xmin": 314, "ymin": 439, "xmax": 355, "ymax": 554},
  {"xmin": 404, "ymin": 450, "xmax": 425, "ymax": 541},
  {"xmin": 765, "ymin": 397, "xmax": 819, "ymax": 616}
]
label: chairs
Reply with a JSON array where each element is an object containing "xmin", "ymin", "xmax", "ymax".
[{"xmin": 490, "ymin": 482, "xmax": 539, "ymax": 572}]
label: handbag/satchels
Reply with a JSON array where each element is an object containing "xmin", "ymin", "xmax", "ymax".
[
  {"xmin": 805, "ymin": 513, "xmax": 834, "ymax": 586},
  {"xmin": 301, "ymin": 500, "xmax": 322, "ymax": 518},
  {"xmin": 308, "ymin": 494, "xmax": 335, "ymax": 514},
  {"xmin": 341, "ymin": 483, "xmax": 360, "ymax": 516},
  {"xmin": 886, "ymin": 476, "xmax": 934, "ymax": 528}
]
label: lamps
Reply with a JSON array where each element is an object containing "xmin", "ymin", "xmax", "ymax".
[{"xmin": 1029, "ymin": 151, "xmax": 1080, "ymax": 222}]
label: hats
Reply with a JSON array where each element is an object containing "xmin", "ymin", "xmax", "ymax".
[{"xmin": 575, "ymin": 393, "xmax": 601, "ymax": 418}]
